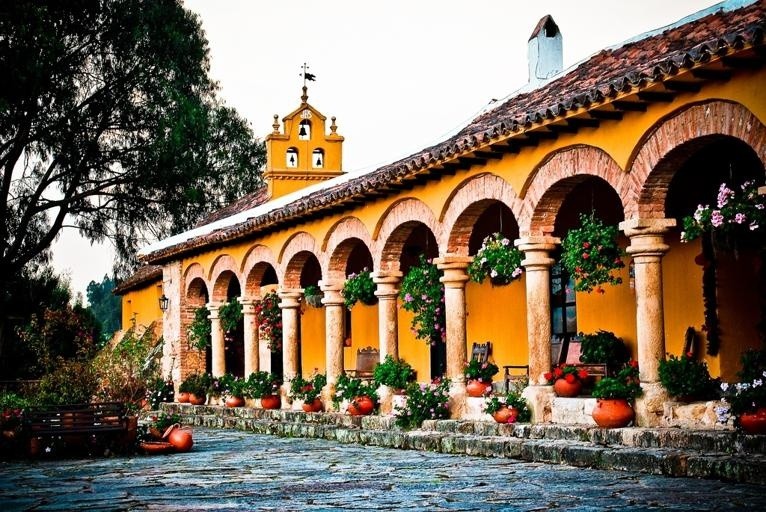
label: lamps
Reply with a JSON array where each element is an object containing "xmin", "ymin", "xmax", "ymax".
[{"xmin": 158, "ymin": 294, "xmax": 170, "ymax": 313}]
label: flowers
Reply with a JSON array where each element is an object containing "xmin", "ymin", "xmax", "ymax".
[
  {"xmin": 183, "ymin": 289, "xmax": 284, "ymax": 360},
  {"xmin": 678, "ymin": 177, "xmax": 765, "ymax": 245},
  {"xmin": 545, "ymin": 348, "xmax": 765, "ymax": 430},
  {"xmin": 563, "ymin": 211, "xmax": 624, "ymax": 296},
  {"xmin": 336, "ymin": 265, "xmax": 376, "ymax": 313},
  {"xmin": 388, "ymin": 381, "xmax": 454, "ymax": 423},
  {"xmin": 401, "ymin": 255, "xmax": 448, "ymax": 348},
  {"xmin": 465, "ymin": 231, "xmax": 524, "ymax": 288}
]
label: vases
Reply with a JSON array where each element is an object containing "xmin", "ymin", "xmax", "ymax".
[
  {"xmin": 360, "ymin": 289, "xmax": 376, "ymax": 307},
  {"xmin": 488, "ymin": 268, "xmax": 510, "ymax": 287},
  {"xmin": 61, "ymin": 414, "xmax": 136, "ymax": 452}
]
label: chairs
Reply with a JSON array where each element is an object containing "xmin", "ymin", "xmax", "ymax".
[
  {"xmin": 345, "ymin": 345, "xmax": 380, "ymax": 386},
  {"xmin": 503, "ymin": 332, "xmax": 565, "ymax": 397}
]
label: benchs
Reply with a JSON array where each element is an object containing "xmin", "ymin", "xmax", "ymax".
[{"xmin": 19, "ymin": 402, "xmax": 130, "ymax": 459}]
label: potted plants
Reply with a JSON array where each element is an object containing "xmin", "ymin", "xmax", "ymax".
[
  {"xmin": 477, "ymin": 390, "xmax": 532, "ymax": 432},
  {"xmin": 460, "ymin": 360, "xmax": 501, "ymax": 400},
  {"xmin": 303, "ymin": 285, "xmax": 324, "ymax": 309},
  {"xmin": 179, "ymin": 368, "xmax": 378, "ymax": 419}
]
